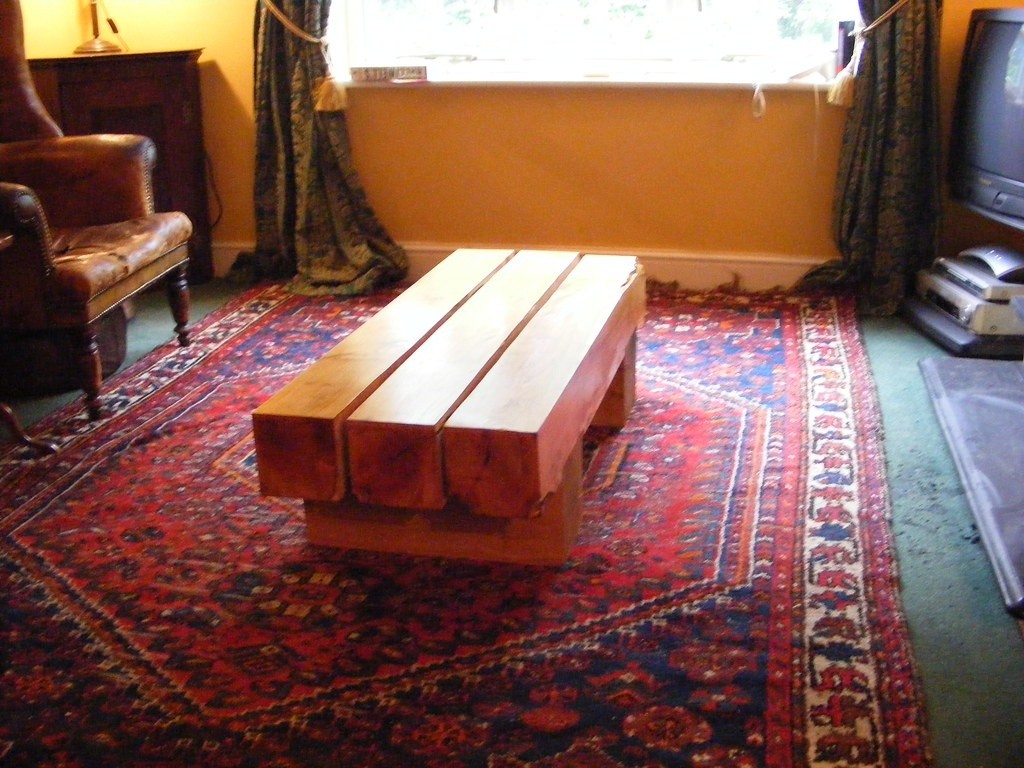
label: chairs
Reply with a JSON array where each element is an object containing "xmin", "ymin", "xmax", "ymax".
[{"xmin": 0, "ymin": 0, "xmax": 192, "ymax": 425}]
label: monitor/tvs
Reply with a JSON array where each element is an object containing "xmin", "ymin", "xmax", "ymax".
[{"xmin": 945, "ymin": 8, "xmax": 1024, "ymax": 219}]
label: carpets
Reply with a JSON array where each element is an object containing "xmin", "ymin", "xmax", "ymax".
[{"xmin": 1, "ymin": 276, "xmax": 933, "ymax": 767}]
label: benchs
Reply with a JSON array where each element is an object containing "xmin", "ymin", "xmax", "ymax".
[{"xmin": 251, "ymin": 244, "xmax": 646, "ymax": 568}]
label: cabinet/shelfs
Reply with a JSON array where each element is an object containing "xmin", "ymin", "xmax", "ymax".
[{"xmin": 21, "ymin": 48, "xmax": 214, "ymax": 284}]
